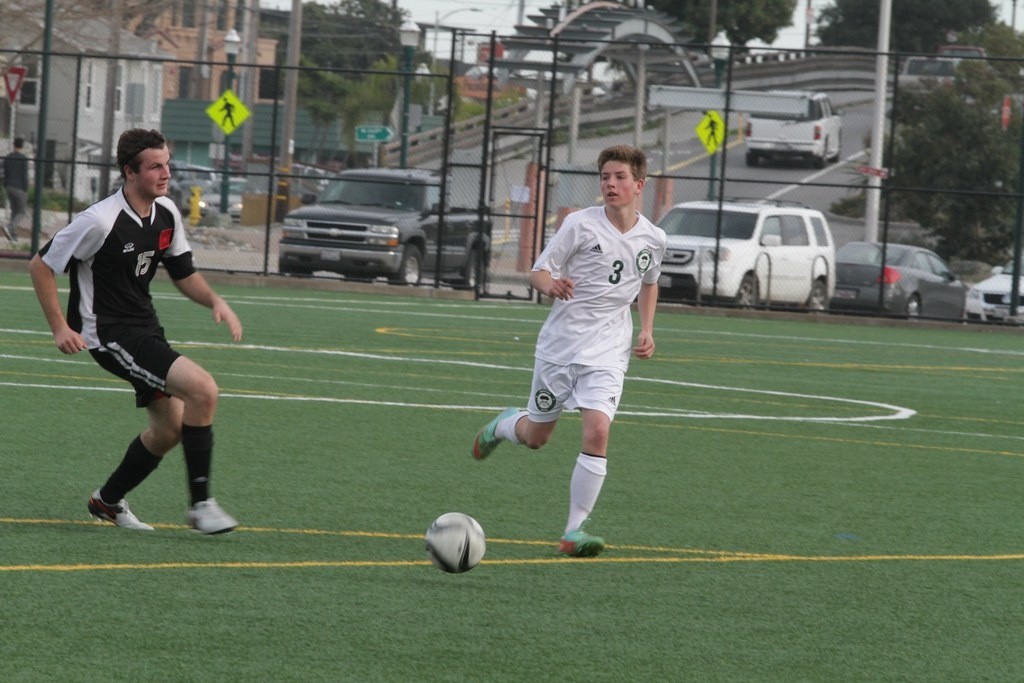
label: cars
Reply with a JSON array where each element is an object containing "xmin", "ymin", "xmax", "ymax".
[
  {"xmin": 964, "ymin": 257, "xmax": 1024, "ymax": 328},
  {"xmin": 197, "ymin": 177, "xmax": 261, "ymax": 221},
  {"xmin": 828, "ymin": 242, "xmax": 971, "ymax": 323},
  {"xmin": 896, "ymin": 57, "xmax": 969, "ymax": 111}
]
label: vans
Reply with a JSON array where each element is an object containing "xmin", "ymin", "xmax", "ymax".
[{"xmin": 937, "ymin": 45, "xmax": 987, "ymax": 88}]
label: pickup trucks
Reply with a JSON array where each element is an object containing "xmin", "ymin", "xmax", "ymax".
[{"xmin": 276, "ymin": 169, "xmax": 493, "ymax": 294}]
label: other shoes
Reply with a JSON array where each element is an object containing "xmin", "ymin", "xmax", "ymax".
[{"xmin": 2, "ymin": 224, "xmax": 18, "ymax": 241}]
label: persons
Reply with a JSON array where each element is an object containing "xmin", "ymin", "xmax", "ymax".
[
  {"xmin": 28, "ymin": 128, "xmax": 243, "ymax": 535},
  {"xmin": 472, "ymin": 145, "xmax": 667, "ymax": 556},
  {"xmin": 0, "ymin": 138, "xmax": 28, "ymax": 242}
]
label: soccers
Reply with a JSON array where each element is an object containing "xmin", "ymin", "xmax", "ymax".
[{"xmin": 425, "ymin": 511, "xmax": 488, "ymax": 575}]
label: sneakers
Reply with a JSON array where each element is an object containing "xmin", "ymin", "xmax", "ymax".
[
  {"xmin": 559, "ymin": 517, "xmax": 605, "ymax": 556},
  {"xmin": 87, "ymin": 488, "xmax": 155, "ymax": 532},
  {"xmin": 472, "ymin": 406, "xmax": 520, "ymax": 460},
  {"xmin": 188, "ymin": 496, "xmax": 240, "ymax": 535}
]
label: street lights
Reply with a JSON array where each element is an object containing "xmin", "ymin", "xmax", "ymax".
[
  {"xmin": 219, "ymin": 29, "xmax": 243, "ymax": 215},
  {"xmin": 428, "ymin": 7, "xmax": 483, "ymax": 118}
]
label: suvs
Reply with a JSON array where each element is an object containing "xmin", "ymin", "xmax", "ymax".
[
  {"xmin": 743, "ymin": 90, "xmax": 845, "ymax": 169},
  {"xmin": 634, "ymin": 197, "xmax": 836, "ymax": 313}
]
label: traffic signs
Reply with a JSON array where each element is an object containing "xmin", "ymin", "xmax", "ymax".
[{"xmin": 354, "ymin": 125, "xmax": 398, "ymax": 142}]
